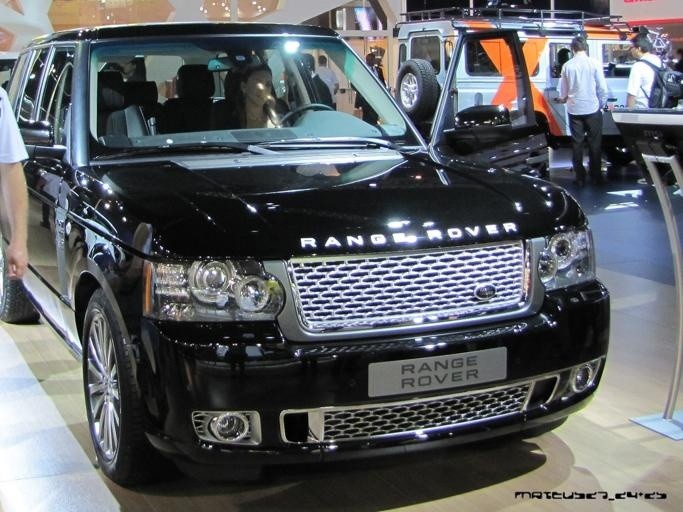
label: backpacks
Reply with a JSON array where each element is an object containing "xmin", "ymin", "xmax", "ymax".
[{"xmin": 637, "ymin": 60, "xmax": 683, "ymax": 108}]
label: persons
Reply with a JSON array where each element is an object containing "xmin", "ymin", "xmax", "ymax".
[
  {"xmin": 316, "ymin": 55, "xmax": 339, "ymax": 111},
  {"xmin": 626, "ymin": 35, "xmax": 665, "ymax": 107},
  {"xmin": 670, "ymin": 48, "xmax": 683, "ymax": 73},
  {"xmin": 0, "ymin": 84, "xmax": 31, "ymax": 279},
  {"xmin": 556, "ymin": 36, "xmax": 609, "ymax": 186},
  {"xmin": 350, "ymin": 53, "xmax": 386, "ymax": 124},
  {"xmin": 302, "ymin": 52, "xmax": 332, "ymax": 106},
  {"xmin": 229, "ymin": 64, "xmax": 294, "ymax": 129}
]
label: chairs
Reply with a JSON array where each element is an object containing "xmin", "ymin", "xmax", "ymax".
[{"xmin": 96, "ymin": 63, "xmax": 296, "ymax": 137}]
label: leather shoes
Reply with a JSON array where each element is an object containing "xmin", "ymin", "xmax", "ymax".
[{"xmin": 576, "ymin": 180, "xmax": 597, "ymax": 186}]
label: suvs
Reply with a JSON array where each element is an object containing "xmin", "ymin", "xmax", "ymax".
[
  {"xmin": 393, "ymin": 5, "xmax": 683, "ymax": 187},
  {"xmin": 0, "ymin": 22, "xmax": 610, "ymax": 485}
]
